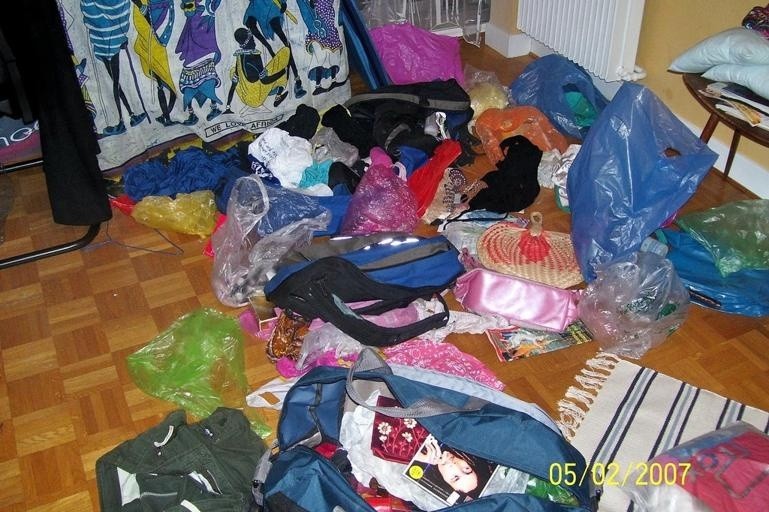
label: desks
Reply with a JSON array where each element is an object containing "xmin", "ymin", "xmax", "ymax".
[{"xmin": 683, "ymin": 72, "xmax": 769, "ymax": 175}]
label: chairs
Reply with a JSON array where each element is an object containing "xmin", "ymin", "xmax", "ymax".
[{"xmin": 0, "ymin": 0, "xmax": 113, "ymax": 269}]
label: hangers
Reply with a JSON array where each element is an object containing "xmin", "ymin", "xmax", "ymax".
[{"xmin": 81, "ymin": 204, "xmax": 184, "ymax": 257}]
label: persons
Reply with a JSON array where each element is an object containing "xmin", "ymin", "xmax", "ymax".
[{"xmin": 415, "ymin": 440, "xmax": 479, "ymax": 495}]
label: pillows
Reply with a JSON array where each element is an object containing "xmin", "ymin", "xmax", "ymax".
[
  {"xmin": 666, "ymin": 27, "xmax": 769, "ymax": 74},
  {"xmin": 700, "ymin": 63, "xmax": 769, "ymax": 100}
]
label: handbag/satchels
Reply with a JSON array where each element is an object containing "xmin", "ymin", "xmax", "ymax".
[
  {"xmin": 510, "ymin": 54, "xmax": 718, "ymax": 283},
  {"xmin": 251, "ymin": 348, "xmax": 602, "ymax": 512},
  {"xmin": 454, "ymin": 268, "xmax": 580, "ymax": 333}
]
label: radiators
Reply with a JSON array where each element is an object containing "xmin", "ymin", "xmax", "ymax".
[{"xmin": 516, "ymin": 0, "xmax": 647, "ymax": 83}]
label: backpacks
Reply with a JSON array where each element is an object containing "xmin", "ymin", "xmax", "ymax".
[{"xmin": 263, "ymin": 231, "xmax": 466, "ymax": 347}]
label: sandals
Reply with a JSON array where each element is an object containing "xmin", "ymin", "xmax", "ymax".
[{"xmin": 103, "ymin": 76, "xmax": 348, "ymax": 134}]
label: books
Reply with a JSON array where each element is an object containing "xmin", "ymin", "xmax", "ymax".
[{"xmin": 698, "ymin": 82, "xmax": 769, "ymax": 131}]
